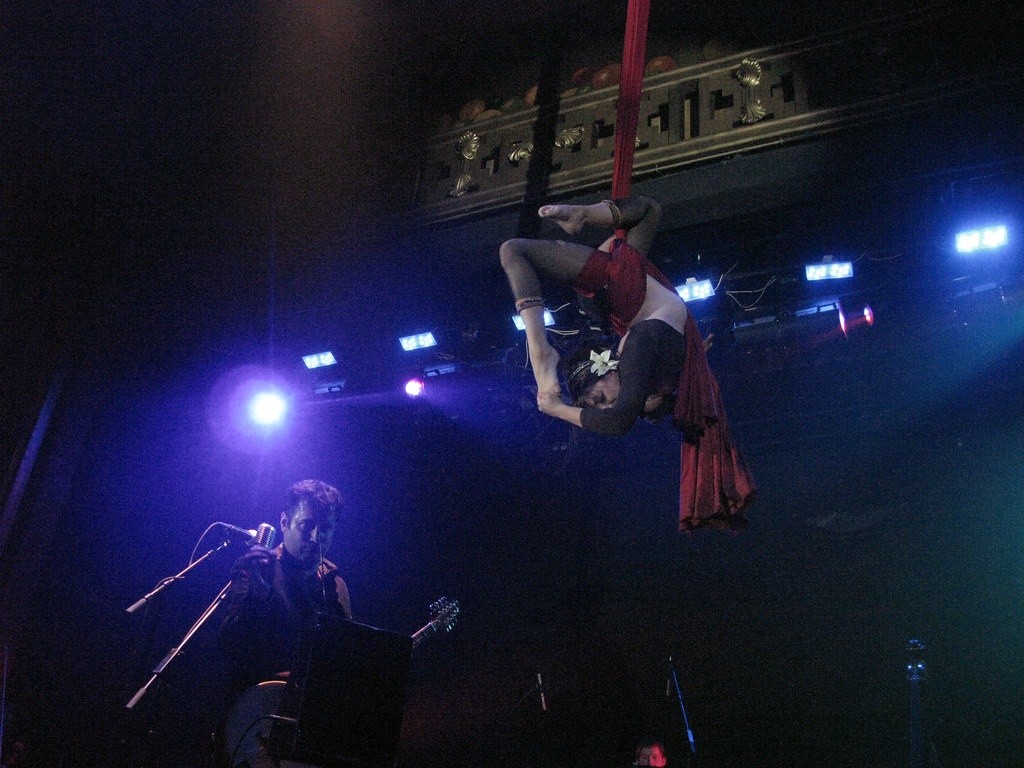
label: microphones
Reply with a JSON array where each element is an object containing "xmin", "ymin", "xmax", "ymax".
[
  {"xmin": 219, "ymin": 522, "xmax": 276, "ymax": 548},
  {"xmin": 666, "ymin": 652, "xmax": 674, "ymax": 696},
  {"xmin": 537, "ymin": 673, "xmax": 547, "ymax": 711}
]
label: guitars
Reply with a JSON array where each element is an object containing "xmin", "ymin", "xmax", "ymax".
[{"xmin": 216, "ymin": 595, "xmax": 460, "ymax": 768}]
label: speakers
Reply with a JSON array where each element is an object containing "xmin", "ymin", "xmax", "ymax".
[{"xmin": 267, "ymin": 612, "xmax": 413, "ymax": 768}]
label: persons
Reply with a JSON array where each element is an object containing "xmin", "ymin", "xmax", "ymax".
[
  {"xmin": 500, "ymin": 194, "xmax": 712, "ymax": 435},
  {"xmin": 218, "ymin": 479, "xmax": 350, "ymax": 680},
  {"xmin": 633, "ymin": 739, "xmax": 667, "ymax": 768}
]
